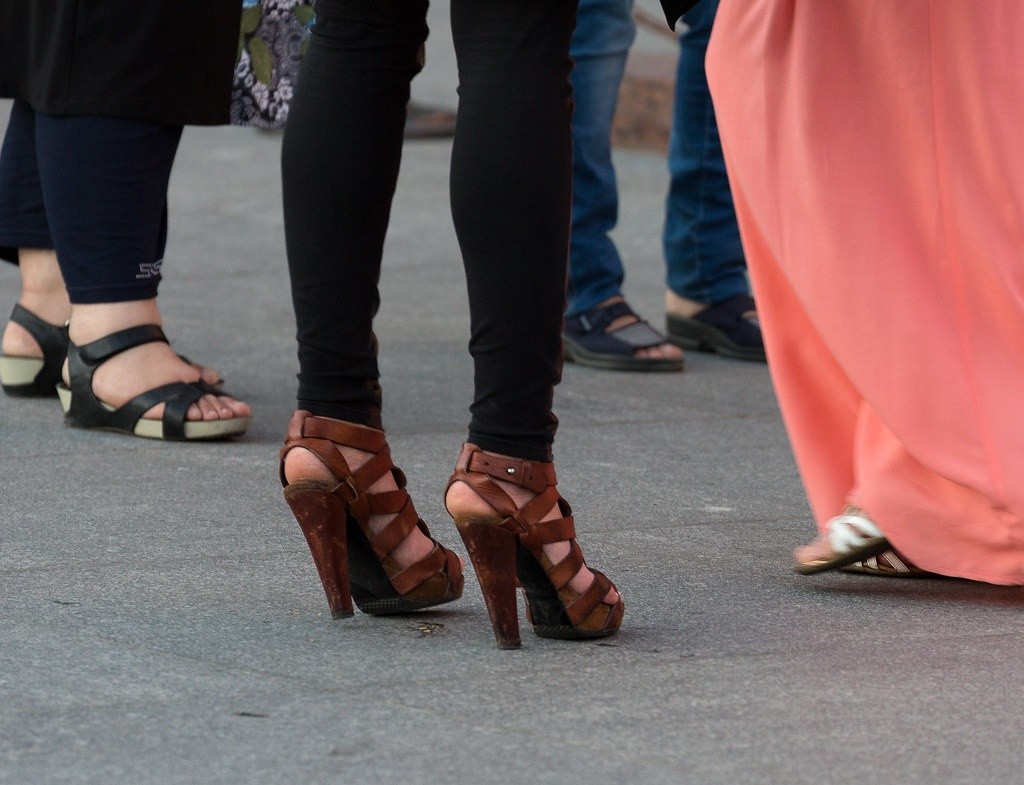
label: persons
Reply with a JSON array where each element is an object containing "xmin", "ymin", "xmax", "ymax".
[
  {"xmin": 703, "ymin": 1, "xmax": 1022, "ymax": 586},
  {"xmin": 0, "ymin": 0, "xmax": 254, "ymax": 446},
  {"xmin": 561, "ymin": 2, "xmax": 768, "ymax": 375},
  {"xmin": 278, "ymin": 0, "xmax": 630, "ymax": 651}
]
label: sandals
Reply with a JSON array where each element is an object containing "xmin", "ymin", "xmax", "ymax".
[
  {"xmin": 793, "ymin": 506, "xmax": 931, "ymax": 579},
  {"xmin": 2, "ymin": 307, "xmax": 251, "ymax": 441},
  {"xmin": 559, "ymin": 298, "xmax": 685, "ymax": 368},
  {"xmin": 665, "ymin": 286, "xmax": 770, "ymax": 362}
]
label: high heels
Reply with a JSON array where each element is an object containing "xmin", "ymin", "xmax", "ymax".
[
  {"xmin": 440, "ymin": 438, "xmax": 627, "ymax": 656},
  {"xmin": 283, "ymin": 406, "xmax": 463, "ymax": 624}
]
